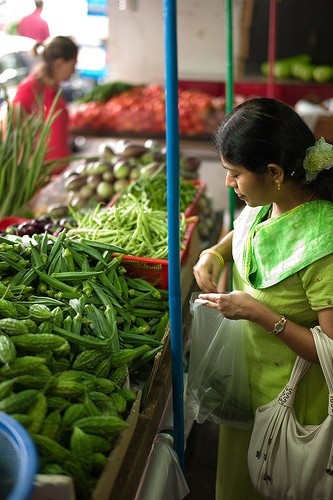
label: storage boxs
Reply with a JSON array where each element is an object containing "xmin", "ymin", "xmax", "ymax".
[
  {"xmin": 106, "ymin": 181, "xmax": 207, "ymax": 217},
  {"xmin": 112, "ymin": 216, "xmax": 200, "ymax": 290}
]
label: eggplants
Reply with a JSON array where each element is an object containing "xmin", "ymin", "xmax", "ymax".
[{"xmin": 5, "ymin": 215, "xmax": 77, "ymax": 238}]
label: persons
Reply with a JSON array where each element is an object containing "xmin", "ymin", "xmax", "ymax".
[
  {"xmin": 16, "ymin": 0, "xmax": 50, "ymax": 44},
  {"xmin": 192, "ymin": 95, "xmax": 333, "ymax": 500},
  {"xmin": 11, "ymin": 35, "xmax": 82, "ymax": 181}
]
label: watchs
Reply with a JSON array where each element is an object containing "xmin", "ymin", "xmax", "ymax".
[{"xmin": 266, "ymin": 315, "xmax": 287, "ymax": 336}]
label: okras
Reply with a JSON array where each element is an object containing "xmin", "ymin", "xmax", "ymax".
[
  {"xmin": 0, "ymin": 228, "xmax": 169, "ymax": 370},
  {"xmin": 197, "ymin": 371, "xmax": 252, "ymax": 421}
]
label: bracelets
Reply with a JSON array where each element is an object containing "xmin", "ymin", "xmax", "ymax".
[{"xmin": 199, "ymin": 249, "xmax": 225, "ymax": 267}]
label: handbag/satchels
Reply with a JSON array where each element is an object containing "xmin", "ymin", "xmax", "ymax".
[{"xmin": 246, "ymin": 325, "xmax": 333, "ymax": 500}]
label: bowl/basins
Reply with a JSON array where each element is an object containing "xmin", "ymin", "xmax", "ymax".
[{"xmin": 0, "ymin": 410, "xmax": 38, "ymax": 500}]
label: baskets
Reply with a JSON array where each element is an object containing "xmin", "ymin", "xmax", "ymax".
[
  {"xmin": 106, "ymin": 215, "xmax": 200, "ymax": 290},
  {"xmin": 104, "ymin": 182, "xmax": 205, "ymax": 218}
]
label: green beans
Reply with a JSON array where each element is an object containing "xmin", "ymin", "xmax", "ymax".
[{"xmin": 65, "ymin": 193, "xmax": 199, "ymax": 286}]
